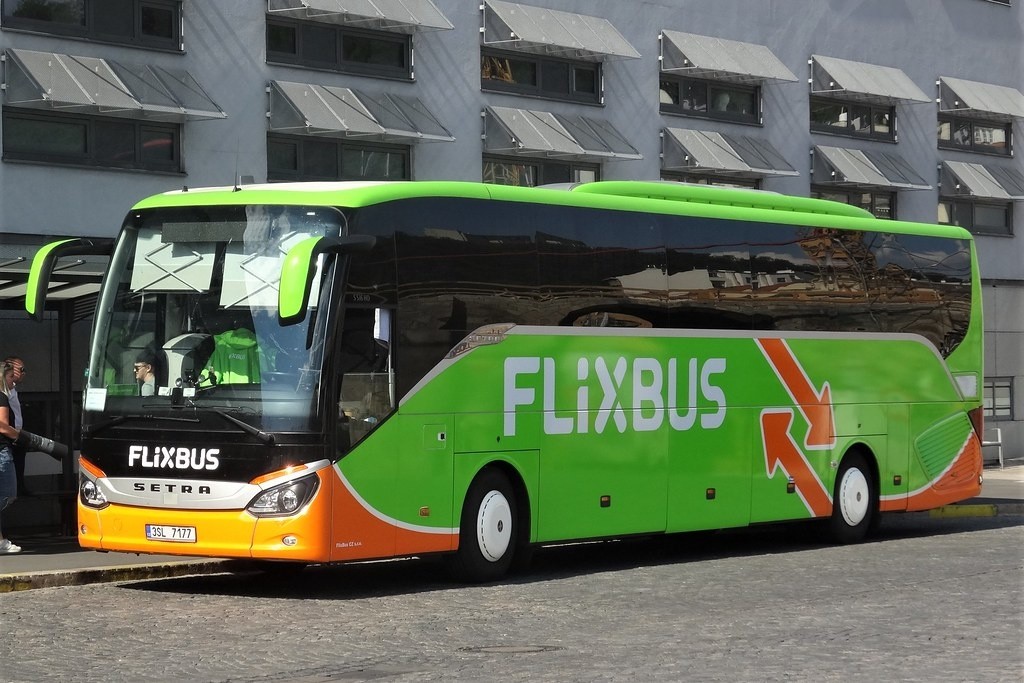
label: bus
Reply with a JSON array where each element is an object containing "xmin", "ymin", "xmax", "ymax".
[{"xmin": 23, "ymin": 177, "xmax": 987, "ymax": 584}]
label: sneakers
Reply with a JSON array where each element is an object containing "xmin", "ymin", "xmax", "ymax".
[{"xmin": 0, "ymin": 539, "xmax": 22, "ymax": 554}]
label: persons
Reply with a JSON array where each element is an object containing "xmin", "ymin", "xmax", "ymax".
[
  {"xmin": 1, "ymin": 356, "xmax": 25, "ymax": 538},
  {"xmin": 196, "ymin": 314, "xmax": 285, "ymax": 388},
  {"xmin": 132, "ymin": 354, "xmax": 159, "ymax": 397},
  {"xmin": 0, "ymin": 362, "xmax": 23, "ymax": 555}
]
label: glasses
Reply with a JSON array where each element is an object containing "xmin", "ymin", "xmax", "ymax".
[
  {"xmin": 135, "ymin": 364, "xmax": 146, "ymax": 372},
  {"xmin": 15, "ymin": 367, "xmax": 27, "ymax": 372}
]
